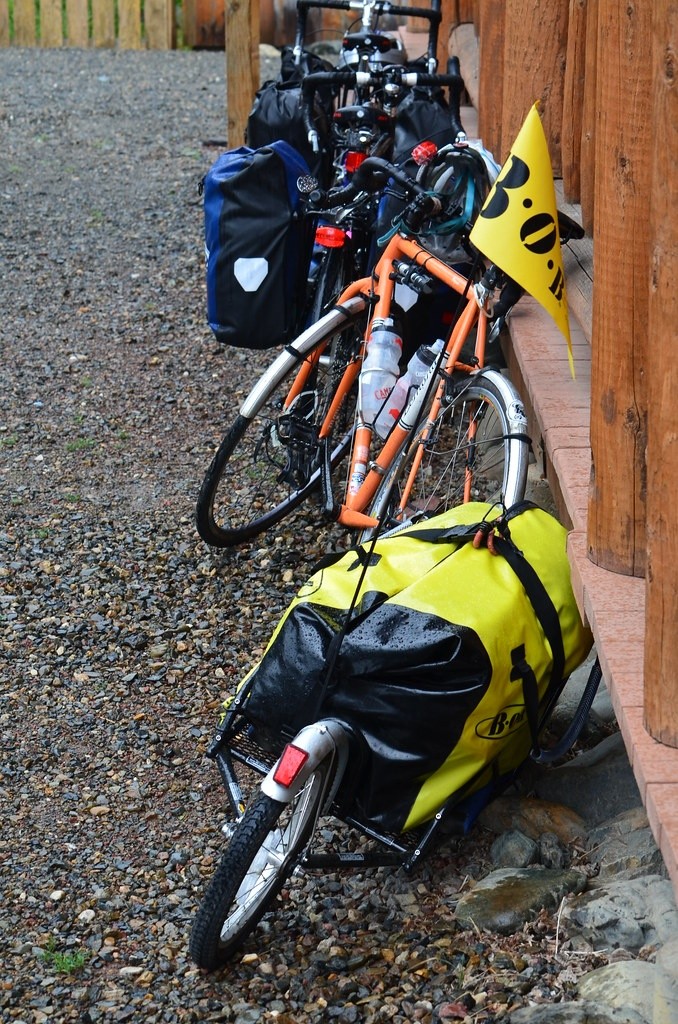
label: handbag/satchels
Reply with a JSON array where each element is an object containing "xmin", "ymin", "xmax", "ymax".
[{"xmin": 251, "ymin": 498, "xmax": 599, "ymax": 837}]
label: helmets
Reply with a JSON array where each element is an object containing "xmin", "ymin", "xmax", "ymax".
[
  {"xmin": 340, "ymin": 30, "xmax": 408, "ymax": 71},
  {"xmin": 412, "ymin": 142, "xmax": 503, "ymax": 266}
]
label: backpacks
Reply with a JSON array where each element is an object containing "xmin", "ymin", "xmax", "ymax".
[
  {"xmin": 200, "ymin": 141, "xmax": 308, "ymax": 350},
  {"xmin": 245, "ymin": 78, "xmax": 304, "ymax": 149}
]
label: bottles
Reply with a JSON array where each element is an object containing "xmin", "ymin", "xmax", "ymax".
[
  {"xmin": 361, "ymin": 318, "xmax": 402, "ymax": 424},
  {"xmin": 374, "ymin": 338, "xmax": 444, "ymax": 440}
]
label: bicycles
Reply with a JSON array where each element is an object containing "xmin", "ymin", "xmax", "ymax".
[{"xmin": 186, "ymin": 0, "xmax": 587, "ymax": 972}]
